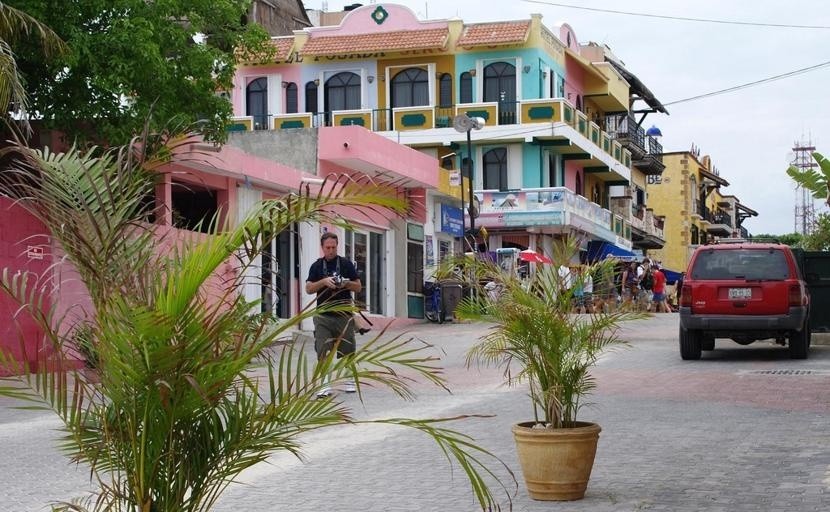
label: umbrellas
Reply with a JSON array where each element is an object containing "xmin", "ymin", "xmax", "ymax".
[{"xmin": 518, "ymin": 246, "xmax": 551, "ymax": 294}]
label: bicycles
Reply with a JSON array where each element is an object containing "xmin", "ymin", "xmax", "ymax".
[{"xmin": 423, "ymin": 276, "xmax": 444, "ymax": 324}]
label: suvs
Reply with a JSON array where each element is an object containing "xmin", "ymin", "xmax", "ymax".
[{"xmin": 678, "ymin": 243, "xmax": 813, "ymax": 361}]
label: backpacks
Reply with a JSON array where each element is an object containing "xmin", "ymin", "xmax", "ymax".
[{"xmin": 640, "ymin": 266, "xmax": 653, "ymax": 290}]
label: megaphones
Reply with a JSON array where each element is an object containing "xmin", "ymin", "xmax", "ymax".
[
  {"xmin": 471, "ymin": 117, "xmax": 485, "ymax": 130},
  {"xmin": 453, "ymin": 114, "xmax": 473, "ymax": 132}
]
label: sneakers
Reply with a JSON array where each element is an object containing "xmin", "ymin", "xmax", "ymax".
[
  {"xmin": 344, "ymin": 384, "xmax": 356, "ymax": 392},
  {"xmin": 318, "ymin": 387, "xmax": 332, "ymax": 397}
]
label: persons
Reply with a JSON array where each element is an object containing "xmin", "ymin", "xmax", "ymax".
[
  {"xmin": 556, "ymin": 253, "xmax": 672, "ymax": 313},
  {"xmin": 482, "ymin": 276, "xmax": 501, "ymax": 302},
  {"xmin": 671, "ymin": 272, "xmax": 687, "ymax": 311},
  {"xmin": 306, "ymin": 229, "xmax": 362, "ymax": 398}
]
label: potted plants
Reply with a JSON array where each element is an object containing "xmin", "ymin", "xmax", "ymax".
[{"xmin": 421, "ymin": 219, "xmax": 656, "ymax": 500}]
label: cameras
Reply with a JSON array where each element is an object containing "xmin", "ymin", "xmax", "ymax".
[{"xmin": 330, "ymin": 271, "xmax": 342, "ymax": 287}]
